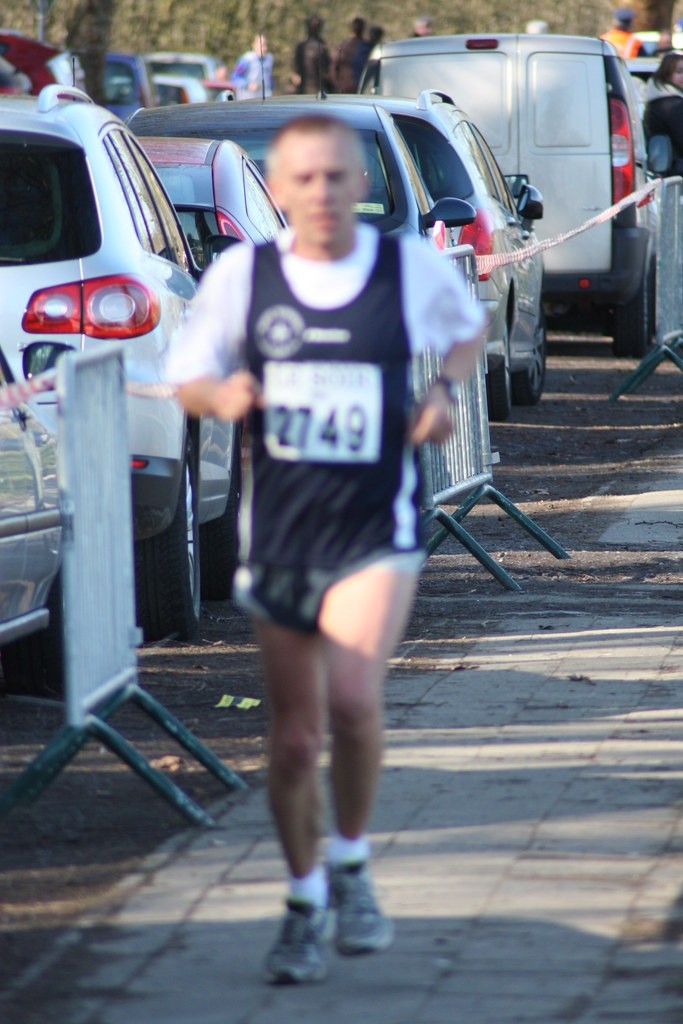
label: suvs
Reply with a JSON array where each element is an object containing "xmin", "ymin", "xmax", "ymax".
[
  {"xmin": 218, "ymin": 88, "xmax": 543, "ymax": 424},
  {"xmin": 0, "ymin": 82, "xmax": 239, "ymax": 647}
]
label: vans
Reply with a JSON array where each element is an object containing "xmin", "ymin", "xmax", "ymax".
[{"xmin": 356, "ymin": 36, "xmax": 656, "ymax": 358}]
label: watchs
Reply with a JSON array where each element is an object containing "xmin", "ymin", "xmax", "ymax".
[{"xmin": 436, "ymin": 375, "xmax": 461, "ymax": 401}]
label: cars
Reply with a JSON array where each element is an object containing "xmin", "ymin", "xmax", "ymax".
[
  {"xmin": 133, "ymin": 134, "xmax": 288, "ymax": 272},
  {"xmin": 0, "ymin": 28, "xmax": 239, "ymax": 119},
  {"xmin": 124, "ymin": 96, "xmax": 476, "ymax": 251},
  {"xmin": 621, "ymin": 29, "xmax": 664, "ymax": 82}
]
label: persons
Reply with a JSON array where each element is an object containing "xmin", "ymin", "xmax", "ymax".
[
  {"xmin": 638, "ymin": 51, "xmax": 683, "ymax": 180},
  {"xmin": 599, "ymin": 8, "xmax": 648, "ymax": 60},
  {"xmin": 291, "ymin": 14, "xmax": 332, "ymax": 94},
  {"xmin": 334, "ymin": 16, "xmax": 386, "ymax": 94},
  {"xmin": 161, "ymin": 110, "xmax": 490, "ymax": 984},
  {"xmin": 411, "ymin": 17, "xmax": 435, "ymax": 39},
  {"xmin": 232, "ymin": 30, "xmax": 276, "ymax": 99}
]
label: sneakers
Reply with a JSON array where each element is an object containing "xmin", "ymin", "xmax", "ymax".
[
  {"xmin": 328, "ymin": 861, "xmax": 390, "ymax": 953},
  {"xmin": 269, "ymin": 896, "xmax": 327, "ymax": 981}
]
